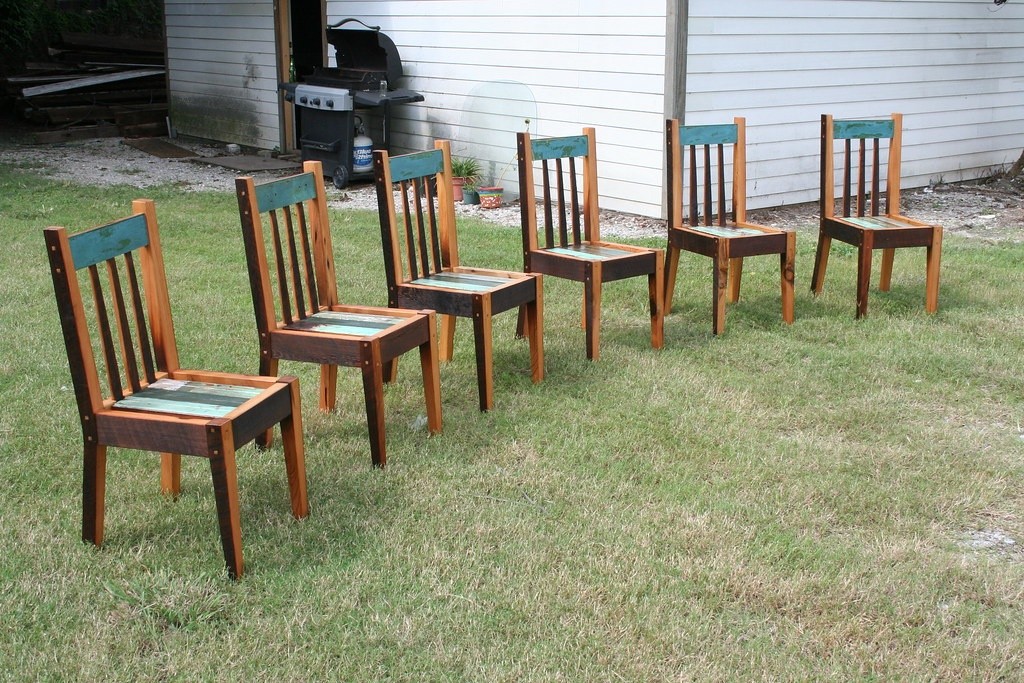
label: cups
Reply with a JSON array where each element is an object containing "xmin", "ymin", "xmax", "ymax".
[{"xmin": 380, "ymin": 81, "xmax": 387, "ymax": 97}]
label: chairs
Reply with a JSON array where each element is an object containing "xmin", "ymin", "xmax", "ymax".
[
  {"xmin": 372, "ymin": 138, "xmax": 545, "ymax": 412},
  {"xmin": 43, "ymin": 199, "xmax": 310, "ymax": 580},
  {"xmin": 235, "ymin": 160, "xmax": 442, "ymax": 470},
  {"xmin": 515, "ymin": 127, "xmax": 663, "ymax": 360},
  {"xmin": 810, "ymin": 112, "xmax": 943, "ymax": 319},
  {"xmin": 663, "ymin": 117, "xmax": 796, "ymax": 337}
]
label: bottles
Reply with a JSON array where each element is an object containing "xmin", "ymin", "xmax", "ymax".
[{"xmin": 289, "ymin": 58, "xmax": 296, "ymax": 84}]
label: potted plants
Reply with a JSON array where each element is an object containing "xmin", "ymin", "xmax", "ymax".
[
  {"xmin": 462, "ymin": 182, "xmax": 482, "ymax": 205},
  {"xmin": 478, "ymin": 119, "xmax": 531, "ymax": 208},
  {"xmin": 430, "ymin": 154, "xmax": 487, "ymax": 200}
]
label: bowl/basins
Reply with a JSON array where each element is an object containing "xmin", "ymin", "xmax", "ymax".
[{"xmin": 479, "ymin": 187, "xmax": 504, "ymax": 208}]
